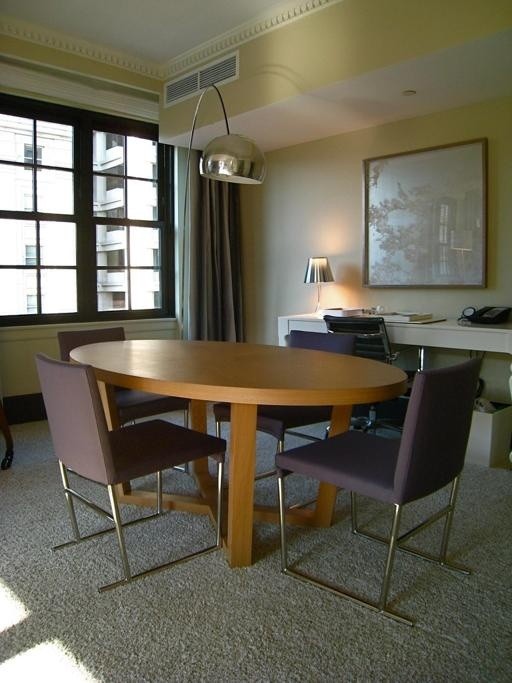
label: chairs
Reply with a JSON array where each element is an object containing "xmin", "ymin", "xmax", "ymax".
[
  {"xmin": 323, "ymin": 315, "xmax": 414, "ymax": 438},
  {"xmin": 275, "ymin": 356, "xmax": 485, "ymax": 627},
  {"xmin": 33, "ymin": 353, "xmax": 227, "ymax": 593},
  {"xmin": 57, "ymin": 327, "xmax": 190, "ymax": 486},
  {"xmin": 213, "ymin": 330, "xmax": 355, "ymax": 509}
]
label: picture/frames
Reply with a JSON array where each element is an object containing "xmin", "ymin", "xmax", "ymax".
[{"xmin": 363, "ymin": 137, "xmax": 489, "ymax": 289}]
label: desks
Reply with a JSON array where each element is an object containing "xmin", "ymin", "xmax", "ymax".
[
  {"xmin": 70, "ymin": 340, "xmax": 408, "ymax": 568},
  {"xmin": 278, "ymin": 311, "xmax": 512, "ymax": 372}
]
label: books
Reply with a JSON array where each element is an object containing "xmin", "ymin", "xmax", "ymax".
[{"xmin": 375, "ymin": 313, "xmax": 434, "ymax": 321}]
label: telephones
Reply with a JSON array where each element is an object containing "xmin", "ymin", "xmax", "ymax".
[{"xmin": 470, "ymin": 306, "xmax": 511, "ymax": 324}]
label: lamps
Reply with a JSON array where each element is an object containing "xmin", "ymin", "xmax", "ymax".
[
  {"xmin": 304, "ymin": 257, "xmax": 334, "ymax": 313},
  {"xmin": 181, "ymin": 85, "xmax": 267, "ymax": 341}
]
